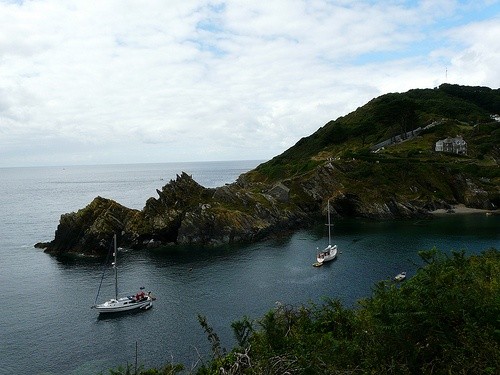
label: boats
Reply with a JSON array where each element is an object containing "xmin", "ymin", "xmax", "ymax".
[{"xmin": 394, "ymin": 271, "xmax": 407, "ymax": 279}]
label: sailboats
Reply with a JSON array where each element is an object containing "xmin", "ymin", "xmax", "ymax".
[
  {"xmin": 316, "ymin": 199, "xmax": 338, "ymax": 264},
  {"xmin": 92, "ymin": 235, "xmax": 156, "ymax": 314}
]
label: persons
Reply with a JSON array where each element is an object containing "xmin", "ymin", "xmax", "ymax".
[
  {"xmin": 136, "ymin": 294, "xmax": 140, "ymax": 300},
  {"xmin": 141, "ymin": 291, "xmax": 145, "ymax": 298}
]
